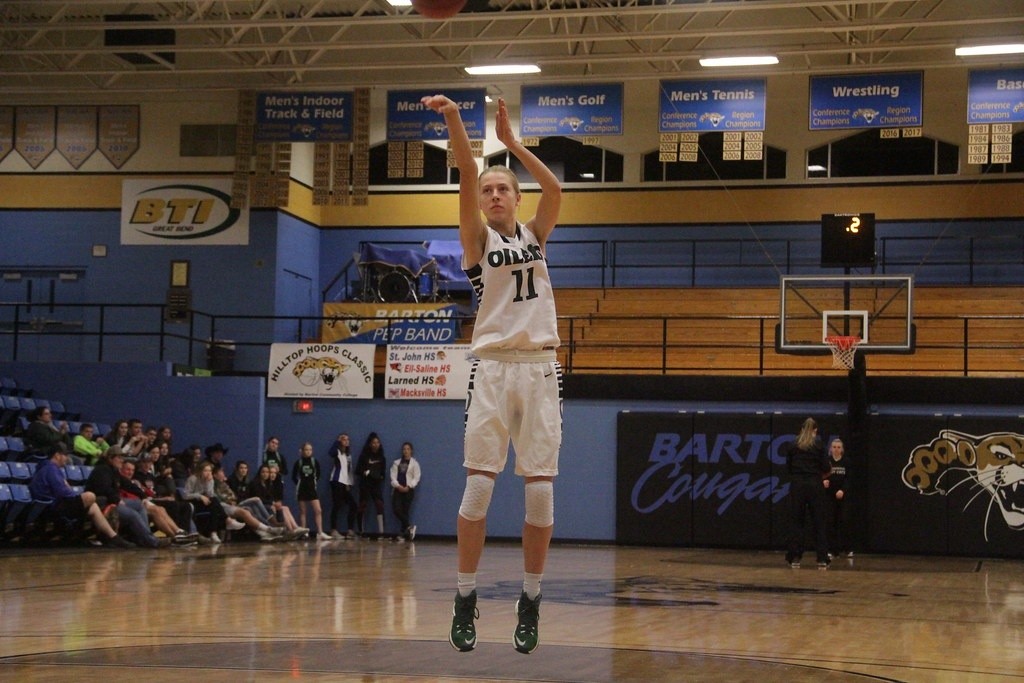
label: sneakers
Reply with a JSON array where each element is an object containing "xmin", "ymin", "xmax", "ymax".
[
  {"xmin": 512, "ymin": 589, "xmax": 542, "ymax": 654},
  {"xmin": 225, "ymin": 519, "xmax": 246, "ymax": 530},
  {"xmin": 449, "ymin": 587, "xmax": 480, "ymax": 652},
  {"xmin": 291, "ymin": 527, "xmax": 310, "ymax": 539},
  {"xmin": 107, "ymin": 534, "xmax": 137, "ymax": 550},
  {"xmin": 317, "ymin": 532, "xmax": 332, "ymax": 540},
  {"xmin": 170, "ymin": 529, "xmax": 200, "ymax": 546},
  {"xmin": 260, "ymin": 527, "xmax": 283, "ymax": 543}
]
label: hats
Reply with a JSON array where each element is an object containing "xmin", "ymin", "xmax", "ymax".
[
  {"xmin": 47, "ymin": 441, "xmax": 75, "ymax": 457},
  {"xmin": 106, "ymin": 445, "xmax": 129, "ymax": 457},
  {"xmin": 205, "ymin": 442, "xmax": 229, "ymax": 456}
]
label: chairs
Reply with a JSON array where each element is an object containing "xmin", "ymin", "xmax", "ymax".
[
  {"xmin": 17, "ymin": 416, "xmax": 30, "ymax": 432},
  {"xmin": 65, "ymin": 465, "xmax": 85, "ymax": 485},
  {"xmin": 53, "ymin": 420, "xmax": 71, "ymax": 433},
  {"xmin": 68, "ymin": 422, "xmax": 83, "ymax": 433},
  {"xmin": 97, "ymin": 424, "xmax": 112, "ymax": 436},
  {"xmin": 50, "ymin": 401, "xmax": 66, "ymax": 420},
  {"xmin": 0, "ymin": 462, "xmax": 11, "ymax": 484},
  {"xmin": 2, "ymin": 376, "xmax": 17, "ymax": 395},
  {"xmin": 90, "ymin": 422, "xmax": 100, "ymax": 436},
  {"xmin": 34, "ymin": 399, "xmax": 52, "ymax": 412},
  {"xmin": 2, "ymin": 395, "xmax": 20, "ymax": 409},
  {"xmin": 8, "ymin": 483, "xmax": 55, "ymax": 524},
  {"xmin": 19, "ymin": 397, "xmax": 36, "ymax": 410},
  {"xmin": 27, "ymin": 462, "xmax": 39, "ymax": 478},
  {"xmin": 0, "ymin": 483, "xmax": 32, "ymax": 525},
  {"xmin": 0, "ymin": 437, "xmax": 10, "ymax": 461},
  {"xmin": 5, "ymin": 437, "xmax": 28, "ymax": 462},
  {"xmin": 80, "ymin": 466, "xmax": 95, "ymax": 481},
  {"xmin": 7, "ymin": 461, "xmax": 31, "ymax": 484}
]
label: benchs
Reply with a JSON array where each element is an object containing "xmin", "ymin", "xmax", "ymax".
[{"xmin": 453, "ymin": 287, "xmax": 1024, "ymax": 379}]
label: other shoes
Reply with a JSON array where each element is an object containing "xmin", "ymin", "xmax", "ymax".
[
  {"xmin": 816, "ymin": 561, "xmax": 827, "ymax": 566},
  {"xmin": 396, "ymin": 535, "xmax": 406, "ymax": 541},
  {"xmin": 211, "ymin": 531, "xmax": 222, "ymax": 543},
  {"xmin": 358, "ymin": 532, "xmax": 370, "ymax": 539},
  {"xmin": 331, "ymin": 531, "xmax": 345, "ymax": 540},
  {"xmin": 827, "ymin": 553, "xmax": 832, "ymax": 556},
  {"xmin": 376, "ymin": 535, "xmax": 385, "ymax": 540},
  {"xmin": 791, "ymin": 558, "xmax": 801, "ymax": 566},
  {"xmin": 345, "ymin": 532, "xmax": 359, "ymax": 539},
  {"xmin": 407, "ymin": 524, "xmax": 416, "ymax": 540},
  {"xmin": 848, "ymin": 551, "xmax": 856, "ymax": 558},
  {"xmin": 158, "ymin": 537, "xmax": 170, "ymax": 548}
]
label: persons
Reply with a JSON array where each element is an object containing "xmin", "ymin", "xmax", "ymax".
[
  {"xmin": 389, "ymin": 439, "xmax": 423, "ymax": 546},
  {"xmin": 785, "ymin": 415, "xmax": 834, "ymax": 573},
  {"xmin": 355, "ymin": 432, "xmax": 388, "ymax": 542},
  {"xmin": 291, "ymin": 440, "xmax": 335, "ymax": 541},
  {"xmin": 30, "ymin": 405, "xmax": 310, "ymax": 550},
  {"xmin": 415, "ymin": 94, "xmax": 564, "ymax": 655},
  {"xmin": 328, "ymin": 433, "xmax": 357, "ymax": 541},
  {"xmin": 821, "ymin": 429, "xmax": 846, "ymax": 559}
]
